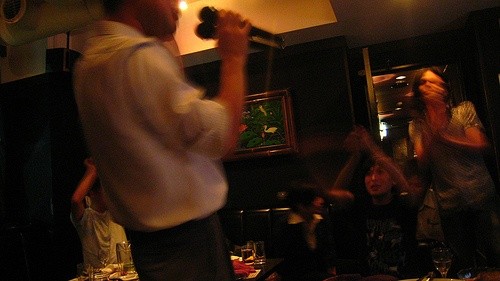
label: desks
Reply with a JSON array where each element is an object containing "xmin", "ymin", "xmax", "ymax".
[{"xmin": 230, "ymin": 258, "xmax": 287, "ymax": 281}]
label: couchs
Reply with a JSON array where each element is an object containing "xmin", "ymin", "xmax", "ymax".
[{"xmin": 218, "ymin": 180, "xmax": 338, "ymax": 281}]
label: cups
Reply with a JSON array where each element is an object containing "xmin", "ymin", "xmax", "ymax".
[
  {"xmin": 241, "ymin": 245, "xmax": 254, "ymax": 265},
  {"xmin": 254, "ymin": 241, "xmax": 266, "ymax": 268},
  {"xmin": 77, "ymin": 263, "xmax": 93, "ymax": 281},
  {"xmin": 116, "ymin": 241, "xmax": 132, "ymax": 276}
]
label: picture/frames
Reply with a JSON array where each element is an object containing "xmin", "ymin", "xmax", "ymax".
[{"xmin": 222, "ymin": 89, "xmax": 300, "ymax": 162}]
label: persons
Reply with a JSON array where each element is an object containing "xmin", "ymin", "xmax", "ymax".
[
  {"xmin": 72, "ymin": 0, "xmax": 252, "ymax": 281},
  {"xmin": 274, "ymin": 180, "xmax": 329, "ymax": 281},
  {"xmin": 65, "ymin": 157, "xmax": 131, "ymax": 273},
  {"xmin": 327, "ymin": 125, "xmax": 418, "ymax": 281},
  {"xmin": 404, "ymin": 67, "xmax": 500, "ymax": 280}
]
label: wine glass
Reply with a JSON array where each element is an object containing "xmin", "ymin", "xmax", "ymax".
[{"xmin": 433, "ymin": 248, "xmax": 452, "ymax": 278}]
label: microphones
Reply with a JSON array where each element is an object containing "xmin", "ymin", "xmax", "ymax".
[{"xmin": 196, "ymin": 7, "xmax": 287, "ymax": 56}]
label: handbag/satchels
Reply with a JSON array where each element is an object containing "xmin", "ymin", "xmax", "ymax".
[{"xmin": 417, "ymin": 186, "xmax": 443, "ymax": 245}]
